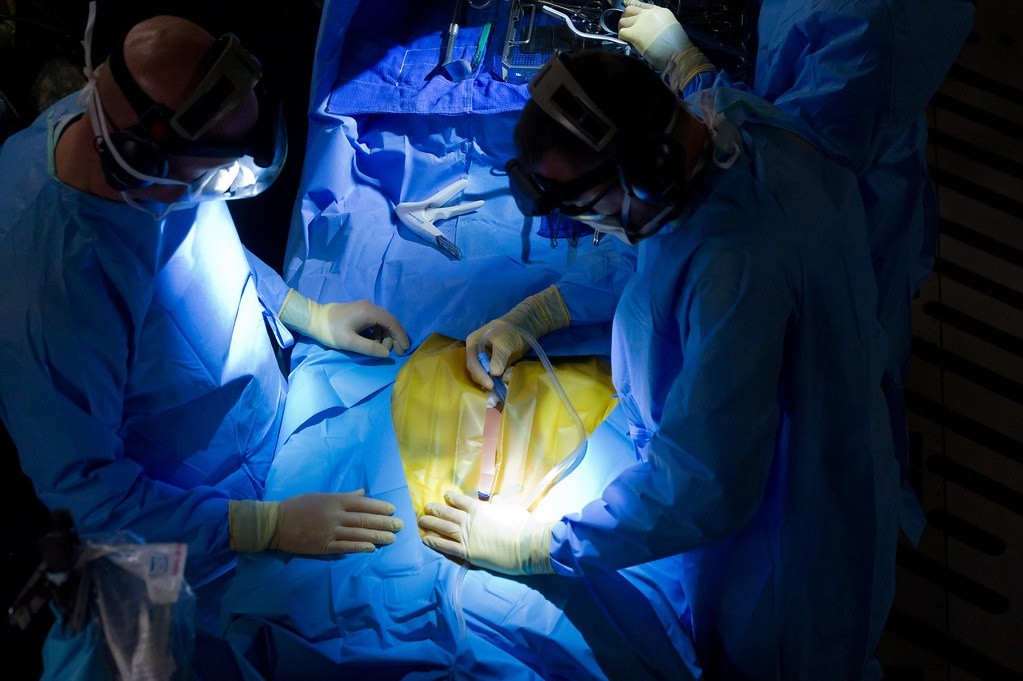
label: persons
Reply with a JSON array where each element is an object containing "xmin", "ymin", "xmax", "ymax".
[
  {"xmin": 618, "ymin": 0, "xmax": 979, "ymax": 588},
  {"xmin": 0, "ymin": 15, "xmax": 409, "ymax": 681},
  {"xmin": 420, "ymin": 50, "xmax": 897, "ymax": 681}
]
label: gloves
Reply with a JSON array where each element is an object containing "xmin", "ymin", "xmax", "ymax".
[
  {"xmin": 466, "ymin": 285, "xmax": 570, "ymax": 391},
  {"xmin": 228, "ymin": 488, "xmax": 402, "ymax": 552},
  {"xmin": 278, "ymin": 288, "xmax": 409, "ymax": 358},
  {"xmin": 418, "ymin": 490, "xmax": 557, "ymax": 576},
  {"xmin": 617, "ymin": 0, "xmax": 717, "ymax": 92}
]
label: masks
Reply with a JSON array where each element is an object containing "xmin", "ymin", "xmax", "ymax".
[
  {"xmin": 579, "ymin": 200, "xmax": 653, "ymax": 247},
  {"xmin": 155, "ymin": 160, "xmax": 239, "ymax": 222}
]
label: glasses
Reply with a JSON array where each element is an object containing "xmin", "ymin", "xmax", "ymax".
[{"xmin": 560, "ymin": 178, "xmax": 618, "ymax": 221}]
